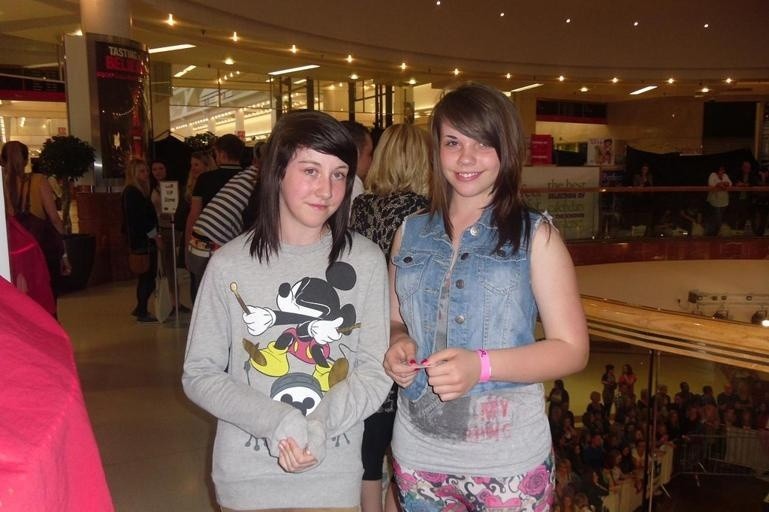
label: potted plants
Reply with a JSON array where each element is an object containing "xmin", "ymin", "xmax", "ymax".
[{"xmin": 37, "ymin": 134, "xmax": 96, "ymax": 293}]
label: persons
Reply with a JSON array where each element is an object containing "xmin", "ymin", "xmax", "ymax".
[
  {"xmin": 352, "ymin": 122, "xmax": 451, "ymax": 512},
  {"xmin": 123, "ymin": 158, "xmax": 160, "ymax": 324},
  {"xmin": 184, "ymin": 131, "xmax": 267, "ymax": 312},
  {"xmin": 592, "ymin": 359, "xmax": 767, "ymax": 511},
  {"xmin": 341, "ymin": 122, "xmax": 376, "ymax": 218},
  {"xmin": 180, "ymin": 110, "xmax": 396, "ymax": 512},
  {"xmin": 544, "ymin": 376, "xmax": 592, "ymax": 511},
  {"xmin": 149, "ymin": 158, "xmax": 189, "ymax": 320},
  {"xmin": 1, "ymin": 140, "xmax": 67, "ymax": 310},
  {"xmin": 383, "ymin": 81, "xmax": 592, "ymax": 512},
  {"xmin": 593, "ymin": 138, "xmax": 769, "ymax": 237}
]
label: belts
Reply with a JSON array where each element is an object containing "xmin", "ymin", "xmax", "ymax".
[{"xmin": 187, "ymin": 245, "xmax": 209, "ymax": 258}]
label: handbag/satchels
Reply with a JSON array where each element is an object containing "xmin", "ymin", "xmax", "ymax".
[
  {"xmin": 127, "ymin": 252, "xmax": 150, "ymax": 274},
  {"xmin": 154, "ymin": 276, "xmax": 173, "ymax": 323}
]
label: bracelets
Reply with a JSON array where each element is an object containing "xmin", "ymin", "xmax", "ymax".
[{"xmin": 477, "ymin": 348, "xmax": 493, "ymax": 384}]
